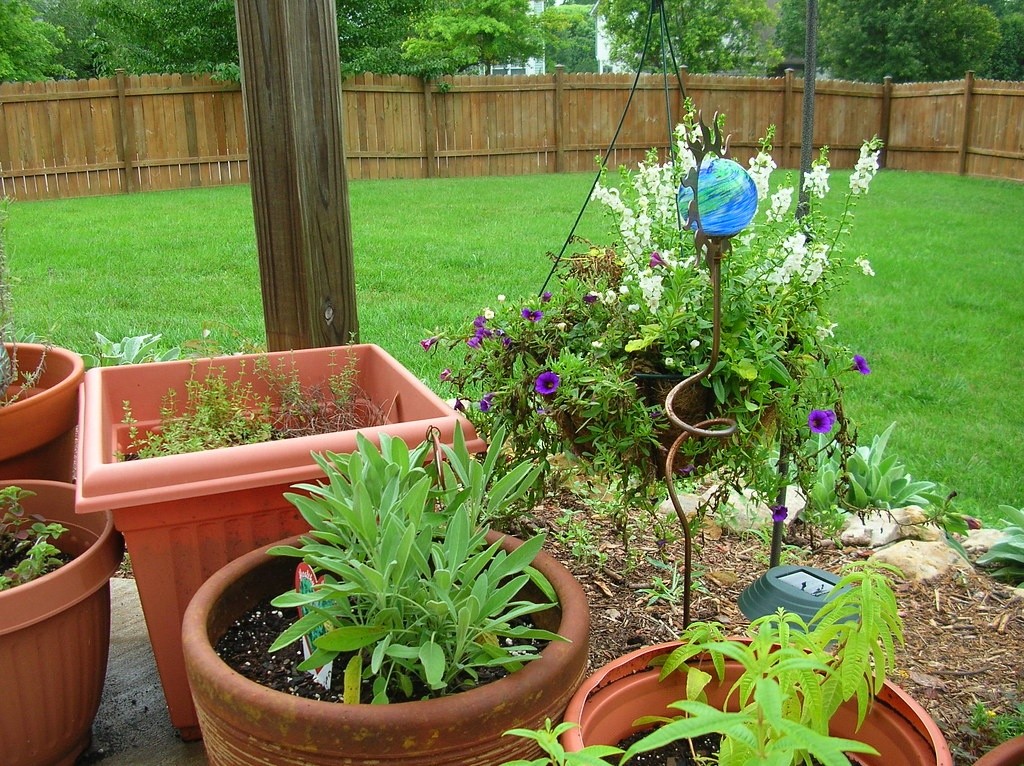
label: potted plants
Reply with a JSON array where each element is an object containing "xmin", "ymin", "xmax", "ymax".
[
  {"xmin": 73, "ymin": 317, "xmax": 486, "ymax": 743},
  {"xmin": 0, "ymin": 194, "xmax": 85, "ymax": 486},
  {"xmin": 0, "ymin": 478, "xmax": 126, "ymax": 766},
  {"xmin": 500, "ymin": 554, "xmax": 951, "ymax": 766},
  {"xmin": 181, "ymin": 419, "xmax": 591, "ymax": 766}
]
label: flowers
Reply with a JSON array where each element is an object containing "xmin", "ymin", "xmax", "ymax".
[{"xmin": 415, "ymin": 98, "xmax": 985, "ymax": 571}]
